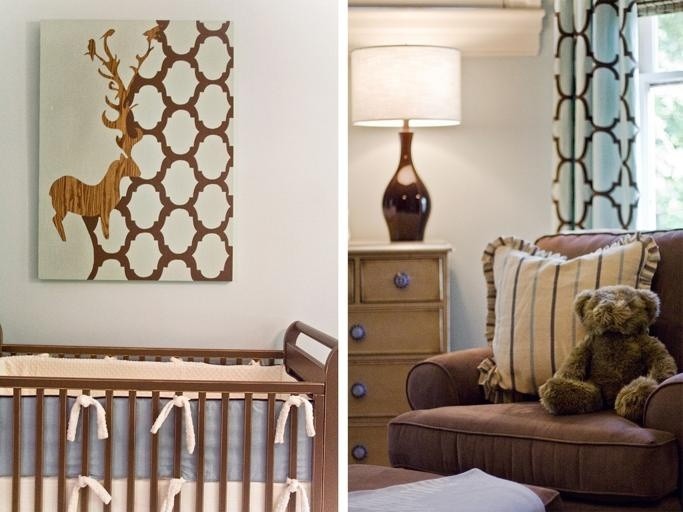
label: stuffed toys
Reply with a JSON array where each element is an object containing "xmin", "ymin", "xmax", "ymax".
[{"xmin": 533, "ymin": 281, "xmax": 678, "ymax": 424}]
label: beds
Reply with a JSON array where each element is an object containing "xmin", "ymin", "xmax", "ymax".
[{"xmin": 0, "ymin": 320, "xmax": 338, "ymax": 512}]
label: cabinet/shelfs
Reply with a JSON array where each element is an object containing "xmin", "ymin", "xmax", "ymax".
[{"xmin": 348, "ymin": 253, "xmax": 449, "ymax": 468}]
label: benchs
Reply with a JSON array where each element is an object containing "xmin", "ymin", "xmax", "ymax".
[{"xmin": 348, "ymin": 462, "xmax": 565, "ymax": 511}]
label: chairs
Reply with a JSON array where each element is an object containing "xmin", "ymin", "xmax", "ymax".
[{"xmin": 386, "ymin": 228, "xmax": 683, "ymax": 512}]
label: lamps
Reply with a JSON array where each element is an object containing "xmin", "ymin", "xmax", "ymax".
[{"xmin": 351, "ymin": 45, "xmax": 460, "ymax": 241}]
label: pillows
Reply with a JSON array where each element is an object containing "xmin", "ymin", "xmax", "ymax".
[{"xmin": 477, "ymin": 232, "xmax": 660, "ymax": 404}]
